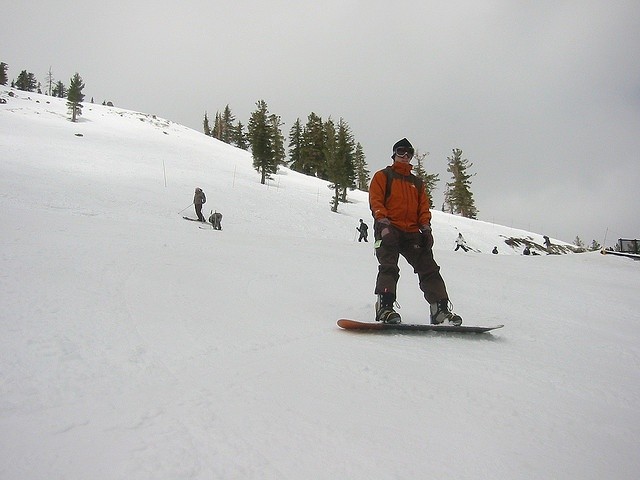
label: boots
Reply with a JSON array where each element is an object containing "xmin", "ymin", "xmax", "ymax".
[
  {"xmin": 430, "ymin": 299, "xmax": 462, "ymax": 325},
  {"xmin": 374, "ymin": 293, "xmax": 400, "ymax": 324}
]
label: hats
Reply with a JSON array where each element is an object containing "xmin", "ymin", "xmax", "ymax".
[{"xmin": 393, "ymin": 138, "xmax": 415, "ymax": 158}]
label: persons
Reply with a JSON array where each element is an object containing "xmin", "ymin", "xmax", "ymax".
[
  {"xmin": 492, "ymin": 246, "xmax": 498, "ymax": 254},
  {"xmin": 356, "ymin": 219, "xmax": 368, "ymax": 242},
  {"xmin": 543, "ymin": 236, "xmax": 551, "ymax": 254},
  {"xmin": 193, "ymin": 188, "xmax": 206, "ymax": 222},
  {"xmin": 209, "ymin": 213, "xmax": 222, "ymax": 230},
  {"xmin": 454, "ymin": 233, "xmax": 467, "ymax": 252},
  {"xmin": 369, "ymin": 138, "xmax": 462, "ymax": 326},
  {"xmin": 523, "ymin": 247, "xmax": 529, "ymax": 255}
]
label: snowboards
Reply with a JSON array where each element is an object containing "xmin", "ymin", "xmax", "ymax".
[{"xmin": 337, "ymin": 319, "xmax": 505, "ymax": 332}]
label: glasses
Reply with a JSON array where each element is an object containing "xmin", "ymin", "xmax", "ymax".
[{"xmin": 395, "ymin": 146, "xmax": 414, "ymax": 158}]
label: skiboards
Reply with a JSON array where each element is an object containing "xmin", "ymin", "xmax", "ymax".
[
  {"xmin": 183, "ymin": 217, "xmax": 208, "ymax": 222},
  {"xmin": 199, "ymin": 226, "xmax": 223, "ymax": 231}
]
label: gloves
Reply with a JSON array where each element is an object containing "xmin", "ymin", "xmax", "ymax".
[
  {"xmin": 421, "ymin": 228, "xmax": 434, "ymax": 249},
  {"xmin": 378, "ymin": 221, "xmax": 398, "ymax": 248}
]
